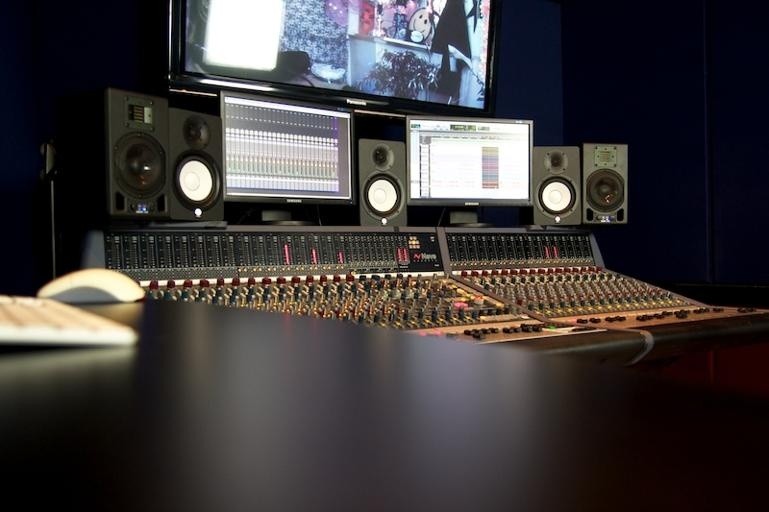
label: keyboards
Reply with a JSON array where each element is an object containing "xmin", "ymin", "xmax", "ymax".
[{"xmin": 0, "ymin": 295, "xmax": 138, "ymax": 348}]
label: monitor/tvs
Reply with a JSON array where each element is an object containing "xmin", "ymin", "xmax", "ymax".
[
  {"xmin": 217, "ymin": 90, "xmax": 357, "ymax": 227},
  {"xmin": 405, "ymin": 115, "xmax": 534, "ymax": 227},
  {"xmin": 168, "ymin": 0, "xmax": 502, "ymax": 120}
]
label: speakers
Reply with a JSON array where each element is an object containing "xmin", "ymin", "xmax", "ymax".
[
  {"xmin": 533, "ymin": 146, "xmax": 581, "ymax": 224},
  {"xmin": 357, "ymin": 138, "xmax": 407, "ymax": 225},
  {"xmin": 168, "ymin": 107, "xmax": 225, "ymax": 222},
  {"xmin": 582, "ymin": 143, "xmax": 630, "ymax": 224},
  {"xmin": 77, "ymin": 88, "xmax": 169, "ymax": 219}
]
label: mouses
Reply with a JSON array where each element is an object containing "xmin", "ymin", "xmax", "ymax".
[{"xmin": 37, "ymin": 268, "xmax": 146, "ymax": 305}]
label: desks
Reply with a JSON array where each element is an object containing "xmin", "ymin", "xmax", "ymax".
[{"xmin": 0, "ymin": 299, "xmax": 765, "ymax": 512}]
